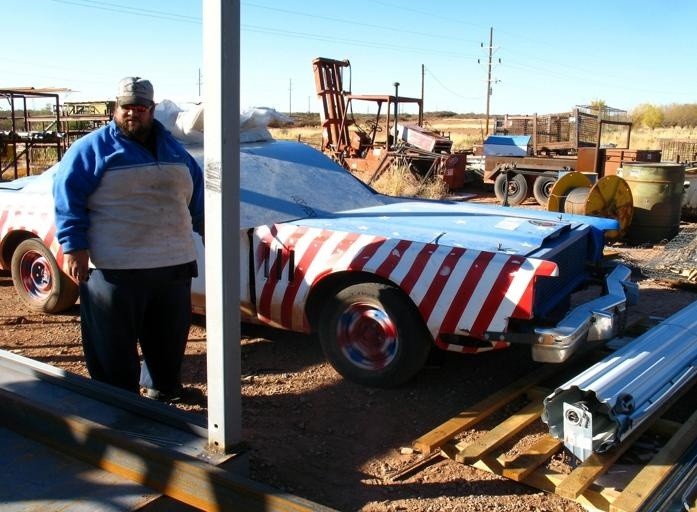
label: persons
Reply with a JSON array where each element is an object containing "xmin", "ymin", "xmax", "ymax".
[{"xmin": 53, "ymin": 76, "xmax": 205, "ymax": 404}]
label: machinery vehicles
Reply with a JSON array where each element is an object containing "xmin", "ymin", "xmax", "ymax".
[
  {"xmin": 484, "ymin": 109, "xmax": 662, "ymax": 206},
  {"xmin": 312, "ymin": 57, "xmax": 475, "ymax": 191}
]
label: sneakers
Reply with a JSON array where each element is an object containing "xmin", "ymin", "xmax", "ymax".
[{"xmin": 146, "ymin": 386, "xmax": 204, "ymax": 404}]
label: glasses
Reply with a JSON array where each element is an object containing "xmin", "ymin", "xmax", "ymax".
[{"xmin": 120, "ymin": 103, "xmax": 152, "ymax": 112}]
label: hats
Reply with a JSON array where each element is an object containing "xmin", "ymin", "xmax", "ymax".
[{"xmin": 115, "ymin": 76, "xmax": 154, "ymax": 109}]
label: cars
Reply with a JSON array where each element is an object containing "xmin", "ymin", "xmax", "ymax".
[{"xmin": 0, "ymin": 106, "xmax": 640, "ymax": 387}]
